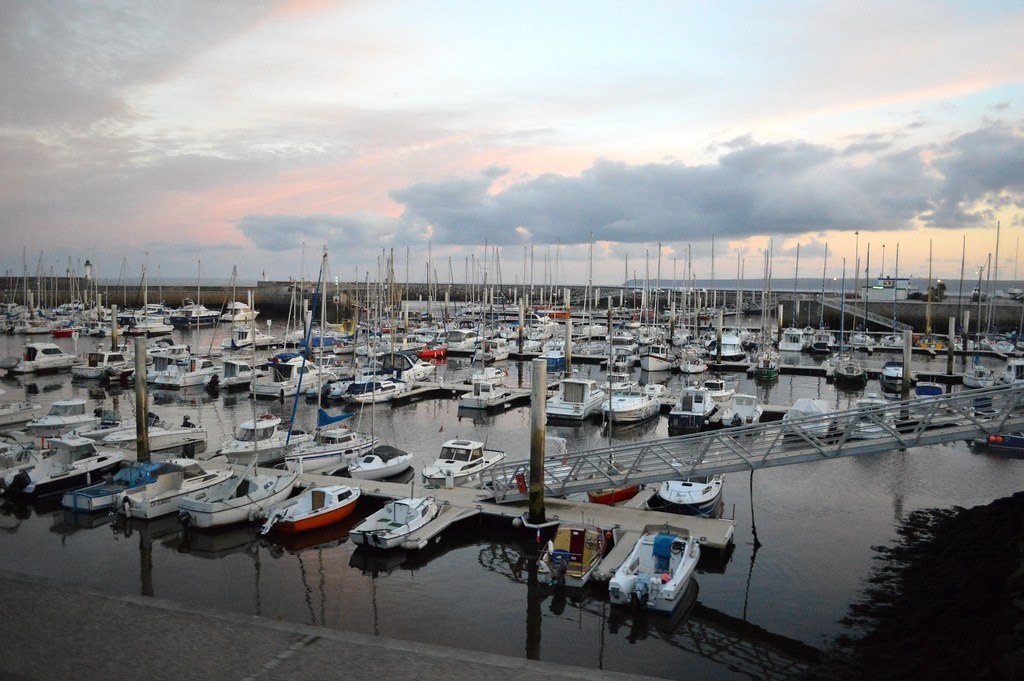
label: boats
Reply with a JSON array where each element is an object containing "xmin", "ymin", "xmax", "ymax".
[
  {"xmin": 421, "ymin": 435, "xmax": 507, "ymax": 487},
  {"xmin": 1, "ymin": 385, "xmax": 309, "ymax": 462},
  {"xmin": 61, "ymin": 460, "xmax": 200, "ymax": 514},
  {"xmin": 607, "ymin": 525, "xmax": 701, "ymax": 608},
  {"xmin": 256, "ymin": 487, "xmax": 361, "ymax": 532},
  {"xmin": 178, "ymin": 470, "xmax": 299, "ymax": 528},
  {"xmin": 496, "ymin": 435, "xmax": 726, "ymax": 516},
  {"xmin": 1, "ymin": 437, "xmax": 126, "ymax": 492},
  {"xmin": 350, "ymin": 480, "xmax": 438, "ymax": 549},
  {"xmin": 538, "ymin": 524, "xmax": 604, "ymax": 588},
  {"xmin": 115, "ymin": 468, "xmax": 238, "ymax": 520}
]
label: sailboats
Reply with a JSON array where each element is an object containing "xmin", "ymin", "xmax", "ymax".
[{"xmin": 1, "ymin": 228, "xmax": 1024, "ymax": 479}]
